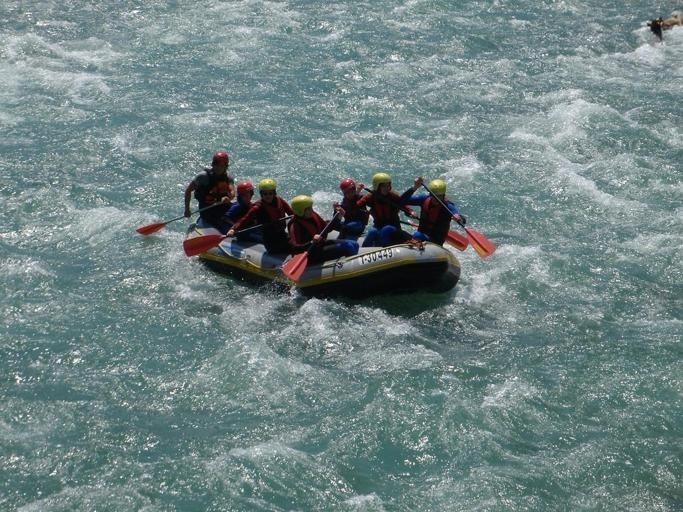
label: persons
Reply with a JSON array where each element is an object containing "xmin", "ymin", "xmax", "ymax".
[
  {"xmin": 325, "ymin": 178, "xmax": 369, "ymax": 240},
  {"xmin": 223, "ymin": 181, "xmax": 264, "ymax": 244},
  {"xmin": 184, "ymin": 151, "xmax": 236, "ymax": 235},
  {"xmin": 352, "ymin": 173, "xmax": 418, "ymax": 248},
  {"xmin": 287, "ymin": 194, "xmax": 359, "ymax": 268},
  {"xmin": 399, "ymin": 176, "xmax": 467, "ymax": 246},
  {"xmin": 227, "ymin": 178, "xmax": 294, "ymax": 257}
]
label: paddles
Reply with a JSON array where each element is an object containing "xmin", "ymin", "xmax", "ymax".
[
  {"xmin": 400, "ymin": 185, "xmax": 469, "ymax": 251},
  {"xmin": 282, "ymin": 209, "xmax": 340, "ymax": 281},
  {"xmin": 183, "ymin": 215, "xmax": 293, "ymax": 257},
  {"xmin": 421, "ymin": 182, "xmax": 497, "ymax": 259},
  {"xmin": 136, "ymin": 202, "xmax": 221, "ymax": 236}
]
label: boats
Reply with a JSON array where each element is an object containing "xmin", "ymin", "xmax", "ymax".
[{"xmin": 183, "ymin": 214, "xmax": 466, "ymax": 299}]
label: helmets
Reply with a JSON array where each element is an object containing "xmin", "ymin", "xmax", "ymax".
[
  {"xmin": 259, "ymin": 178, "xmax": 277, "ymax": 191},
  {"xmin": 428, "ymin": 179, "xmax": 447, "ymax": 195},
  {"xmin": 236, "ymin": 180, "xmax": 254, "ymax": 195},
  {"xmin": 290, "ymin": 194, "xmax": 313, "ymax": 217},
  {"xmin": 340, "ymin": 178, "xmax": 356, "ymax": 194},
  {"xmin": 372, "ymin": 173, "xmax": 392, "ymax": 191},
  {"xmin": 212, "ymin": 152, "xmax": 229, "ymax": 166}
]
